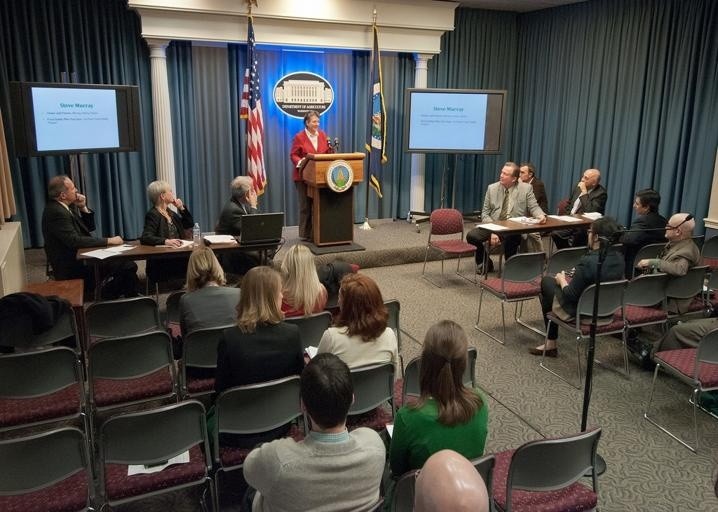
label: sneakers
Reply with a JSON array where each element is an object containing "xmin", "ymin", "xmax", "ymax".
[{"xmin": 477, "ymin": 260, "xmax": 494, "ymax": 275}]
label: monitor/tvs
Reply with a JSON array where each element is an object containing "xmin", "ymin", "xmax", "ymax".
[
  {"xmin": 9, "ymin": 80, "xmax": 142, "ymax": 158},
  {"xmin": 403, "ymin": 87, "xmax": 507, "ymax": 155}
]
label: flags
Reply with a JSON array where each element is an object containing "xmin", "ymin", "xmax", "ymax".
[
  {"xmin": 365, "ymin": 23, "xmax": 388, "ymax": 199},
  {"xmin": 240, "ymin": 14, "xmax": 268, "ymax": 198}
]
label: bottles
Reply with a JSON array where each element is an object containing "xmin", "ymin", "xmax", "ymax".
[
  {"xmin": 192, "ymin": 222, "xmax": 201, "ymax": 247},
  {"xmin": 416, "ymin": 224, "xmax": 420, "ymax": 233},
  {"xmin": 410, "ymin": 215, "xmax": 412, "ymax": 224}
]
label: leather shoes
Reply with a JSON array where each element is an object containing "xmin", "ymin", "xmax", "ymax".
[
  {"xmin": 528, "ymin": 348, "xmax": 557, "ymax": 357},
  {"xmin": 628, "ymin": 337, "xmax": 651, "ymax": 363}
]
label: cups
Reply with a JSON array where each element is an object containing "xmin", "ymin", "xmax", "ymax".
[{"xmin": 412, "ymin": 217, "xmax": 416, "ymax": 224}]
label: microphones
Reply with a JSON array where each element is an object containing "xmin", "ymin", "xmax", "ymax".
[
  {"xmin": 602, "ymin": 213, "xmax": 694, "ymax": 261},
  {"xmin": 334, "ymin": 137, "xmax": 340, "ymax": 149},
  {"xmin": 327, "ymin": 137, "xmax": 331, "ymax": 149}
]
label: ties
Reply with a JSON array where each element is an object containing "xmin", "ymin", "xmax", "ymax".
[{"xmin": 500, "ymin": 190, "xmax": 509, "ymax": 220}]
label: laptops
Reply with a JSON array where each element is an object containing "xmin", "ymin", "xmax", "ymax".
[{"xmin": 234, "ymin": 213, "xmax": 284, "ymax": 244}]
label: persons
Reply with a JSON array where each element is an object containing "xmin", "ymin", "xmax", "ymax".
[
  {"xmin": 551, "ymin": 168, "xmax": 608, "ymax": 249},
  {"xmin": 381, "ymin": 322, "xmax": 490, "ymax": 512},
  {"xmin": 624, "ymin": 316, "xmax": 717, "ymax": 367},
  {"xmin": 303, "ymin": 272, "xmax": 397, "ymax": 424},
  {"xmin": 200, "ymin": 265, "xmax": 302, "ymax": 450},
  {"xmin": 243, "ymin": 352, "xmax": 388, "ymax": 512},
  {"xmin": 41, "ymin": 175, "xmax": 140, "ymax": 300},
  {"xmin": 291, "ymin": 110, "xmax": 336, "ymax": 242},
  {"xmin": 139, "ymin": 179, "xmax": 194, "ymax": 292},
  {"xmin": 617, "ymin": 187, "xmax": 669, "ymax": 279},
  {"xmin": 628, "ymin": 213, "xmax": 699, "ymax": 314},
  {"xmin": 414, "ymin": 447, "xmax": 488, "ymax": 512},
  {"xmin": 173, "ymin": 245, "xmax": 227, "ymax": 379},
  {"xmin": 214, "ymin": 175, "xmax": 274, "ymax": 284},
  {"xmin": 281, "ymin": 244, "xmax": 329, "ymax": 317},
  {"xmin": 529, "ymin": 215, "xmax": 625, "ymax": 355},
  {"xmin": 466, "ymin": 161, "xmax": 548, "ymax": 273}
]
